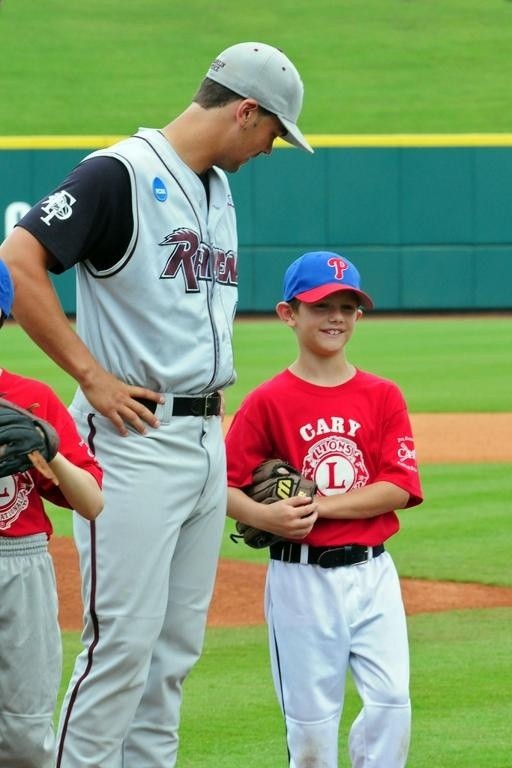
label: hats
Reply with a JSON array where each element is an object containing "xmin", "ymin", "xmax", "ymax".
[
  {"xmin": 206, "ymin": 39, "xmax": 314, "ymax": 156},
  {"xmin": 283, "ymin": 250, "xmax": 374, "ymax": 314}
]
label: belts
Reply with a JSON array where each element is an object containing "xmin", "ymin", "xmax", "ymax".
[
  {"xmin": 270, "ymin": 539, "xmax": 386, "ymax": 568},
  {"xmin": 131, "ymin": 386, "xmax": 222, "ymax": 416}
]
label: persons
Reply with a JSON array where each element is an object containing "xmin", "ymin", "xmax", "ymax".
[
  {"xmin": 1, "ymin": 40, "xmax": 315, "ymax": 768},
  {"xmin": 0, "ymin": 257, "xmax": 106, "ymax": 767},
  {"xmin": 225, "ymin": 249, "xmax": 425, "ymax": 768}
]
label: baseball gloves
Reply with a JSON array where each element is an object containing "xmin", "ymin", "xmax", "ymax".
[
  {"xmin": 1, "ymin": 398, "xmax": 59, "ymax": 488},
  {"xmin": 232, "ymin": 461, "xmax": 318, "ymax": 549}
]
